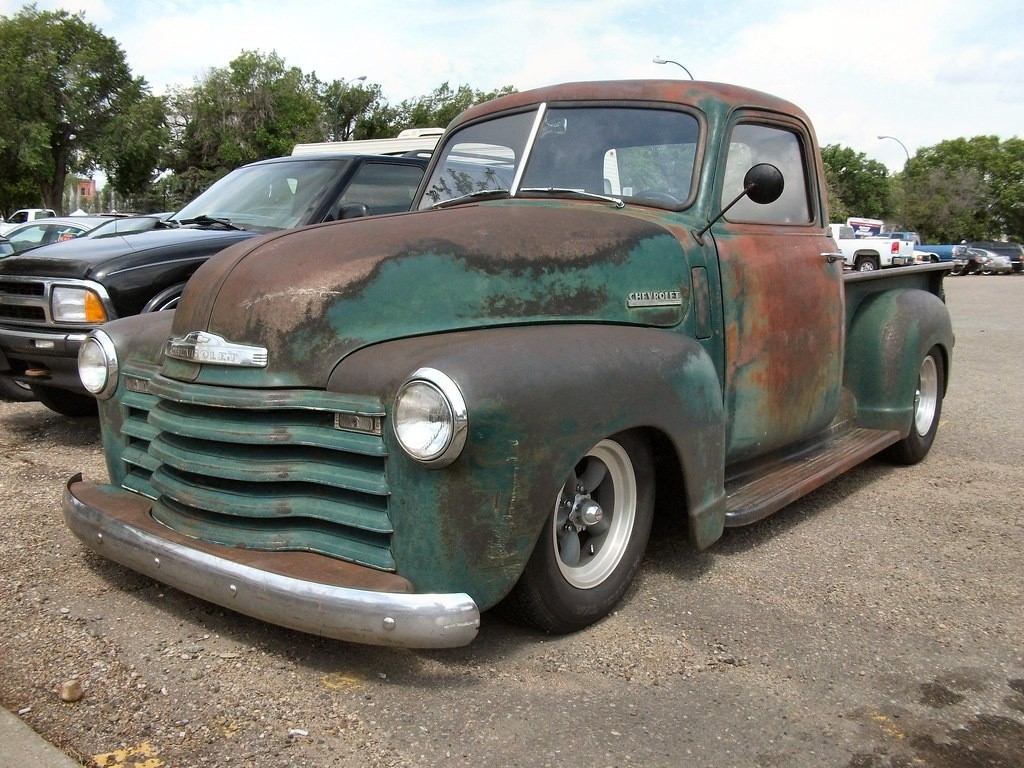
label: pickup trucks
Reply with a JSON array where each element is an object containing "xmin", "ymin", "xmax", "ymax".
[
  {"xmin": 874, "ymin": 232, "xmax": 969, "ymax": 276},
  {"xmin": 825, "ymin": 224, "xmax": 916, "ymax": 272}
]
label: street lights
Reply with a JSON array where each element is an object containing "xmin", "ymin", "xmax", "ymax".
[{"xmin": 332, "ymin": 75, "xmax": 366, "ymax": 141}]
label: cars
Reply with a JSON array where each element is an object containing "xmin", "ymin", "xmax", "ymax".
[
  {"xmin": 0, "ymin": 117, "xmax": 507, "ymax": 428},
  {"xmin": 963, "ymin": 242, "xmax": 1024, "ymax": 275},
  {"xmin": 58, "ymin": 76, "xmax": 955, "ymax": 650}
]
